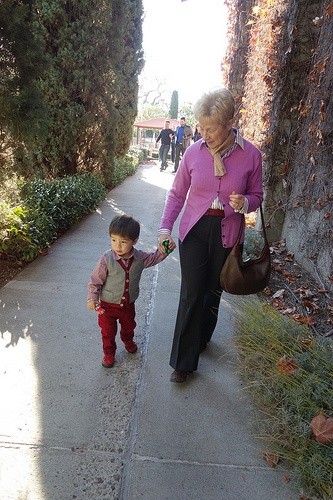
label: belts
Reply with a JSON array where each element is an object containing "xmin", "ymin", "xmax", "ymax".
[{"xmin": 205, "ymin": 208, "xmax": 224, "ymax": 217}]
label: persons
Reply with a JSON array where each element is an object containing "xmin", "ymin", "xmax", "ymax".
[
  {"xmin": 172, "ymin": 117, "xmax": 193, "ymax": 173},
  {"xmin": 157, "ymin": 117, "xmax": 174, "ymax": 175},
  {"xmin": 194, "ymin": 124, "xmax": 203, "ymax": 142},
  {"xmin": 157, "ymin": 90, "xmax": 264, "ymax": 382},
  {"xmin": 87, "ymin": 215, "xmax": 176, "ymax": 368}
]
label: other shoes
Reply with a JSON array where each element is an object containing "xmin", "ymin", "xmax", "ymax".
[
  {"xmin": 102, "ymin": 353, "xmax": 115, "ymax": 368},
  {"xmin": 170, "ymin": 370, "xmax": 186, "ymax": 382},
  {"xmin": 123, "ymin": 339, "xmax": 137, "ymax": 354}
]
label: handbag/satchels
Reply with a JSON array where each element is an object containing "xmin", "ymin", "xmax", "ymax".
[{"xmin": 219, "ymin": 194, "xmax": 271, "ymax": 296}]
